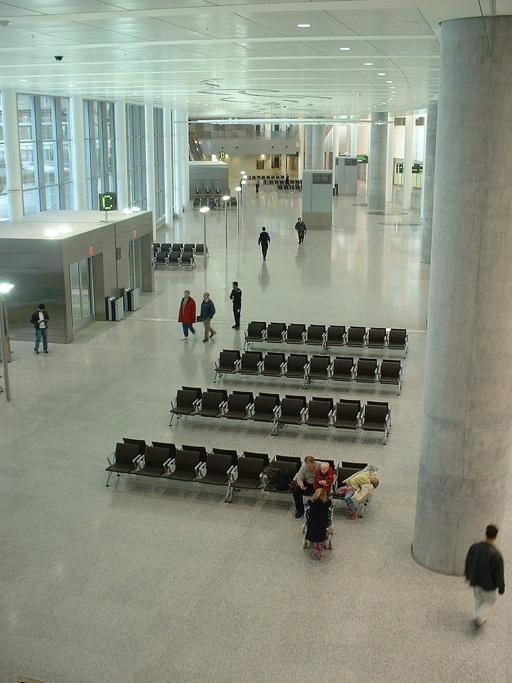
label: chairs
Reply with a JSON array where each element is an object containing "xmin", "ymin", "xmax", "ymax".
[
  {"xmin": 167, "ymin": 318, "xmax": 410, "ymax": 442},
  {"xmin": 190, "ymin": 187, "xmax": 239, "ymax": 210},
  {"xmin": 151, "ymin": 241, "xmax": 210, "ymax": 271},
  {"xmin": 247, "ymin": 174, "xmax": 303, "ymax": 191},
  {"xmin": 98, "ymin": 435, "xmax": 377, "ymax": 519}
]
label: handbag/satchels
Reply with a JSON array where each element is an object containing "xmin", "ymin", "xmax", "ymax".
[{"xmin": 264, "ymin": 465, "xmax": 292, "ymax": 491}]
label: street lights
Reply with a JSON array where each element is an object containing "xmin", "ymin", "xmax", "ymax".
[
  {"xmin": 200, "ymin": 205, "xmax": 210, "ymax": 268},
  {"xmin": 1, "ymin": 281, "xmax": 15, "ymax": 400},
  {"xmin": 222, "ymin": 195, "xmax": 230, "ymax": 250},
  {"xmin": 234, "ymin": 185, "xmax": 241, "ymax": 235},
  {"xmin": 240, "ymin": 170, "xmax": 247, "ymax": 223}
]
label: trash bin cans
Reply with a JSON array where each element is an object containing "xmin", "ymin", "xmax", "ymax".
[
  {"xmin": 333, "ymin": 184, "xmax": 338, "ymax": 196},
  {"xmin": 105, "ymin": 297, "xmax": 124, "ymax": 323},
  {"xmin": 123, "ymin": 288, "xmax": 139, "ymax": 312}
]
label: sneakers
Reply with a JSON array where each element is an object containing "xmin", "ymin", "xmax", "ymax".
[
  {"xmin": 203, "ymin": 338, "xmax": 209, "ymax": 342},
  {"xmin": 209, "ymin": 332, "xmax": 216, "ymax": 337},
  {"xmin": 181, "ymin": 337, "xmax": 188, "ymax": 342},
  {"xmin": 33, "ymin": 349, "xmax": 39, "ymax": 354},
  {"xmin": 43, "ymin": 347, "xmax": 48, "ymax": 353},
  {"xmin": 232, "ymin": 322, "xmax": 241, "ymax": 330},
  {"xmin": 293, "ymin": 507, "xmax": 304, "ymax": 518}
]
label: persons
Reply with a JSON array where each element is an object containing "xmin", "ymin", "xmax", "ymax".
[
  {"xmin": 463, "ymin": 524, "xmax": 504, "ymax": 632},
  {"xmin": 29, "ymin": 303, "xmax": 50, "ymax": 354},
  {"xmin": 200, "ymin": 291, "xmax": 217, "ymax": 342},
  {"xmin": 177, "ymin": 290, "xmax": 196, "ymax": 342},
  {"xmin": 291, "ymin": 456, "xmax": 379, "ymax": 562},
  {"xmin": 286, "ymin": 173, "xmax": 290, "ymax": 185},
  {"xmin": 258, "ymin": 226, "xmax": 271, "ymax": 261},
  {"xmin": 230, "ymin": 281, "xmax": 242, "ymax": 330},
  {"xmin": 255, "ymin": 177, "xmax": 260, "ymax": 193},
  {"xmin": 295, "ymin": 218, "xmax": 307, "ymax": 244}
]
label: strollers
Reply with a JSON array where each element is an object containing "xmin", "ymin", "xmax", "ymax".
[{"xmin": 302, "ymin": 499, "xmax": 335, "ymax": 551}]
